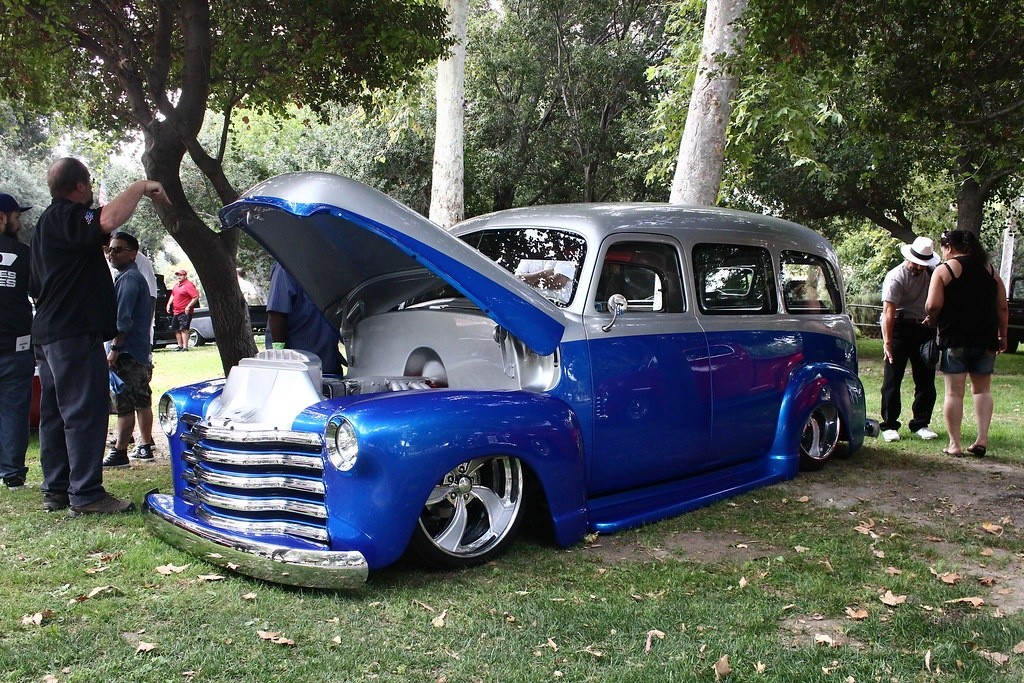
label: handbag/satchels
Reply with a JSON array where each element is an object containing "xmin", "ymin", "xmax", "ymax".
[{"xmin": 919, "ymin": 340, "xmax": 940, "ymax": 370}]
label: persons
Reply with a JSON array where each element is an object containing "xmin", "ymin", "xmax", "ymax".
[
  {"xmin": 103, "ymin": 228, "xmax": 157, "ymax": 468},
  {"xmin": 879, "ymin": 237, "xmax": 943, "ymax": 441},
  {"xmin": 0, "ymin": 193, "xmax": 36, "ymax": 488},
  {"xmin": 265, "ymin": 260, "xmax": 348, "ymax": 377},
  {"xmin": 236, "ymin": 267, "xmax": 260, "ymax": 304},
  {"xmin": 514, "ymin": 259, "xmax": 575, "ymax": 304},
  {"xmin": 166, "ymin": 269, "xmax": 199, "ymax": 352},
  {"xmin": 27, "ymin": 156, "xmax": 172, "ymax": 515},
  {"xmin": 922, "ymin": 229, "xmax": 1009, "ymax": 457}
]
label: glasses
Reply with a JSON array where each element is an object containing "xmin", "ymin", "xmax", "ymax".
[
  {"xmin": 104, "ymin": 246, "xmax": 132, "ymax": 253},
  {"xmin": 942, "ymin": 231, "xmax": 947, "ymax": 239}
]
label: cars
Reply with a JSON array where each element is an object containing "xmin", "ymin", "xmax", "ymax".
[
  {"xmin": 998, "ymin": 275, "xmax": 1024, "ymax": 354},
  {"xmin": 133, "ymin": 168, "xmax": 869, "ymax": 590}
]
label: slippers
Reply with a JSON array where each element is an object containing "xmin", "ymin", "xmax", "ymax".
[
  {"xmin": 966, "ymin": 443, "xmax": 986, "ymax": 457},
  {"xmin": 943, "ymin": 447, "xmax": 964, "ymax": 457}
]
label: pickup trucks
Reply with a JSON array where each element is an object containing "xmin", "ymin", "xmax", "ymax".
[{"xmin": 150, "ymin": 273, "xmax": 270, "ymax": 351}]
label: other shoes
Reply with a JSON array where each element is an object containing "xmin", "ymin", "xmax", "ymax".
[
  {"xmin": 133, "ymin": 437, "xmax": 155, "ymax": 451},
  {"xmin": 1, "ymin": 475, "xmax": 25, "ymax": 490},
  {"xmin": 173, "ymin": 346, "xmax": 183, "ymax": 351},
  {"xmin": 106, "ymin": 435, "xmax": 135, "ymax": 445},
  {"xmin": 180, "ymin": 348, "xmax": 188, "ymax": 352}
]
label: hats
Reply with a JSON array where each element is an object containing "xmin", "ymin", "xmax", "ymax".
[
  {"xmin": 175, "ymin": 270, "xmax": 187, "ymax": 275},
  {"xmin": 0, "ymin": 192, "xmax": 32, "ymax": 212},
  {"xmin": 901, "ymin": 236, "xmax": 941, "ymax": 267}
]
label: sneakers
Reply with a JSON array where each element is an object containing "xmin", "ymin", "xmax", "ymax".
[
  {"xmin": 882, "ymin": 429, "xmax": 900, "ymax": 442},
  {"xmin": 43, "ymin": 493, "xmax": 69, "ymax": 511},
  {"xmin": 128, "ymin": 442, "xmax": 155, "ymax": 462},
  {"xmin": 70, "ymin": 490, "xmax": 135, "ymax": 513},
  {"xmin": 102, "ymin": 446, "xmax": 130, "ymax": 468},
  {"xmin": 916, "ymin": 426, "xmax": 939, "ymax": 440}
]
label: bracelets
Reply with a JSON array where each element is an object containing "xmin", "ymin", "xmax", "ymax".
[{"xmin": 187, "ymin": 305, "xmax": 191, "ymax": 308}]
